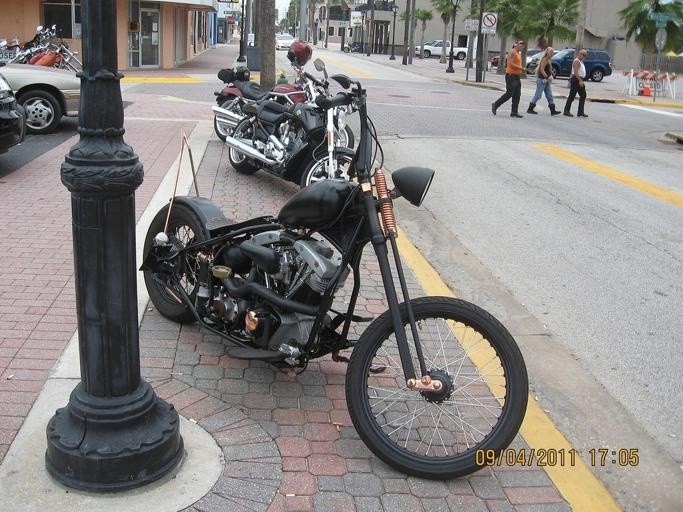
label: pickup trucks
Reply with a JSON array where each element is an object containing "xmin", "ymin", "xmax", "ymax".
[{"xmin": 415, "ymin": 40, "xmax": 468, "ymax": 60}]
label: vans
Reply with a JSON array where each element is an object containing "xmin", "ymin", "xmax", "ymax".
[{"xmin": 491, "ymin": 49, "xmax": 612, "ymax": 82}]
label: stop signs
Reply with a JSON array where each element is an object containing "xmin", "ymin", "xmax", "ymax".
[{"xmin": 481, "ymin": 12, "xmax": 498, "ymax": 29}]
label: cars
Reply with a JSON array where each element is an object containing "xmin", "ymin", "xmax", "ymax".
[
  {"xmin": 276, "ymin": 35, "xmax": 295, "ymax": 50},
  {"xmin": 0, "ymin": 60, "xmax": 81, "ymax": 153}
]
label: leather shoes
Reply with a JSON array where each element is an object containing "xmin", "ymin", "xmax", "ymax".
[
  {"xmin": 577, "ymin": 113, "xmax": 588, "ymax": 117},
  {"xmin": 492, "ymin": 103, "xmax": 497, "ymax": 115},
  {"xmin": 511, "ymin": 112, "xmax": 523, "ymax": 118},
  {"xmin": 564, "ymin": 111, "xmax": 573, "ymax": 116}
]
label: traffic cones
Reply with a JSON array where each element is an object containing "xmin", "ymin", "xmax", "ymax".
[{"xmin": 642, "ymin": 78, "xmax": 651, "ymax": 97}]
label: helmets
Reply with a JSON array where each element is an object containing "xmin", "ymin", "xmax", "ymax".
[{"xmin": 287, "ymin": 40, "xmax": 312, "ymax": 65}]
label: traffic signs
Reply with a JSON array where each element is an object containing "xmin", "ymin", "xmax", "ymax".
[{"xmin": 648, "ymin": 13, "xmax": 673, "ymax": 27}]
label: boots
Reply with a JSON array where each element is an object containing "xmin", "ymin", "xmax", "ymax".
[
  {"xmin": 548, "ymin": 104, "xmax": 561, "ymax": 115},
  {"xmin": 527, "ymin": 102, "xmax": 537, "ymax": 114}
]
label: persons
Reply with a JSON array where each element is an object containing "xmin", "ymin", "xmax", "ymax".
[
  {"xmin": 563, "ymin": 50, "xmax": 588, "ymax": 117},
  {"xmin": 492, "ymin": 41, "xmax": 527, "ymax": 118},
  {"xmin": 527, "ymin": 47, "xmax": 560, "ymax": 116}
]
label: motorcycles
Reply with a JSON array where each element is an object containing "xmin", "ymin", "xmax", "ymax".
[
  {"xmin": 0, "ymin": 25, "xmax": 82, "ymax": 73},
  {"xmin": 343, "ymin": 41, "xmax": 363, "ymax": 53}
]
